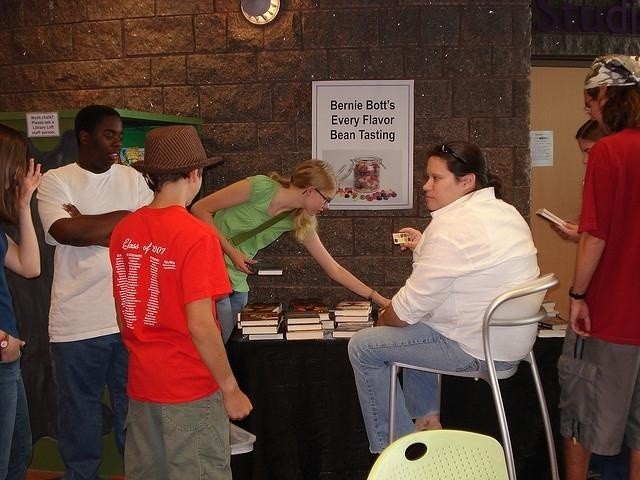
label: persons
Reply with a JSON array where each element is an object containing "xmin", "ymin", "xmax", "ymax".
[
  {"xmin": 190, "ymin": 159, "xmax": 392, "ymax": 343},
  {"xmin": 1, "ymin": 124, "xmax": 43, "ymax": 480},
  {"xmin": 110, "ymin": 124, "xmax": 254, "ymax": 480},
  {"xmin": 558, "ymin": 54, "xmax": 640, "ymax": 480},
  {"xmin": 549, "ymin": 116, "xmax": 609, "ymax": 243},
  {"xmin": 37, "ymin": 106, "xmax": 155, "ymax": 480},
  {"xmin": 348, "ymin": 143, "xmax": 541, "ymax": 456}
]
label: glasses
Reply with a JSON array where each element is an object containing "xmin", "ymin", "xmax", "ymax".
[
  {"xmin": 440, "ymin": 143, "xmax": 482, "ymax": 174},
  {"xmin": 583, "ymin": 96, "xmax": 595, "ymax": 114},
  {"xmin": 302, "ymin": 187, "xmax": 330, "ymax": 205}
]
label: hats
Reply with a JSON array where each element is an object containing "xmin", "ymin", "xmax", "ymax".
[
  {"xmin": 128, "ymin": 124, "xmax": 223, "ymax": 175},
  {"xmin": 583, "ymin": 53, "xmax": 640, "ymax": 91}
]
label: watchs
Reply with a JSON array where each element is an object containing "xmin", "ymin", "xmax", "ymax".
[
  {"xmin": 1, "ymin": 333, "xmax": 9, "ymax": 352},
  {"xmin": 568, "ymin": 288, "xmax": 587, "ymax": 299}
]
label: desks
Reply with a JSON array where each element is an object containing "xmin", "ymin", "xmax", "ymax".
[{"xmin": 224, "ymin": 325, "xmax": 630, "ymax": 480}]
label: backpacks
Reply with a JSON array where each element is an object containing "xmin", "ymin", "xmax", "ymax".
[{"xmin": 226, "ymin": 209, "xmax": 301, "ymax": 249}]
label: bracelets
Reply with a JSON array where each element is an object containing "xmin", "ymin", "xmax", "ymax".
[{"xmin": 369, "ymin": 290, "xmax": 377, "ymax": 303}]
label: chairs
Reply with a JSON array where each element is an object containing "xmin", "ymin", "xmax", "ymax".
[
  {"xmin": 388, "ymin": 273, "xmax": 559, "ymax": 480},
  {"xmin": 367, "ymin": 430, "xmax": 510, "ymax": 480}
]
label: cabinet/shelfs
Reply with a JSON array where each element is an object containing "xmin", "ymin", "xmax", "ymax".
[{"xmin": 0, "ymin": 110, "xmax": 202, "ymax": 164}]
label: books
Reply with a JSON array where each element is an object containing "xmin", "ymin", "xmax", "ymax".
[
  {"xmin": 242, "ymin": 302, "xmax": 283, "ymax": 321},
  {"xmin": 285, "ymin": 332, "xmax": 324, "ymax": 341},
  {"xmin": 320, "ymin": 321, "xmax": 334, "ymax": 330},
  {"xmin": 286, "ymin": 312, "xmax": 320, "ymax": 324},
  {"xmin": 246, "ymin": 264, "xmax": 282, "ymax": 277},
  {"xmin": 535, "ymin": 211, "xmax": 562, "ymax": 226},
  {"xmin": 334, "ymin": 300, "xmax": 373, "ymax": 316},
  {"xmin": 333, "ymin": 321, "xmax": 374, "ymax": 338},
  {"xmin": 334, "ymin": 314, "xmax": 371, "ymax": 323},
  {"xmin": 240, "ymin": 315, "xmax": 283, "ymax": 327},
  {"xmin": 242, "ymin": 323, "xmax": 282, "ymax": 334},
  {"xmin": 287, "ymin": 324, "xmax": 322, "ymax": 331},
  {"xmin": 249, "ymin": 329, "xmax": 284, "ymax": 340},
  {"xmin": 319, "ymin": 312, "xmax": 330, "ymax": 321},
  {"xmin": 539, "ymin": 207, "xmax": 569, "ymax": 227}
]
